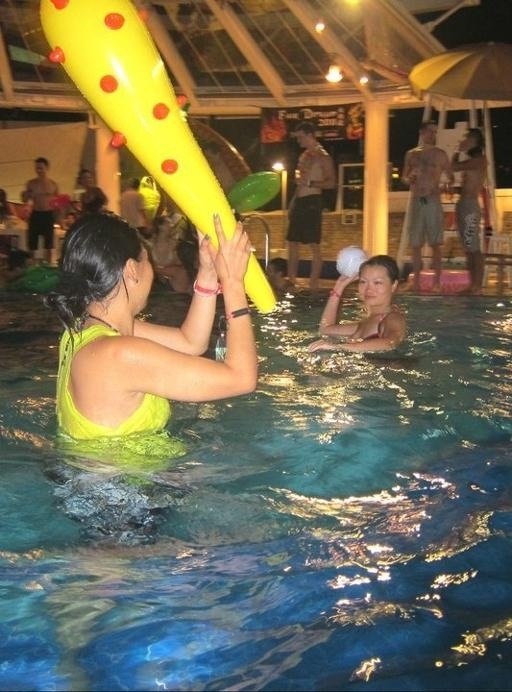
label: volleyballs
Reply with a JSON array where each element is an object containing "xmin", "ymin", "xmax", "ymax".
[{"xmin": 336, "ymin": 243, "xmax": 367, "ymax": 276}]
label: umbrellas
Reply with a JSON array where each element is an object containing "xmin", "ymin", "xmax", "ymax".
[{"xmin": 407, "ymin": 39, "xmax": 511, "ymax": 103}]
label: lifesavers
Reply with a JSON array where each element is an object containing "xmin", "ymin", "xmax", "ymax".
[
  {"xmin": 406, "ymin": 269, "xmax": 471, "ymax": 298},
  {"xmin": 4, "ymin": 264, "xmax": 60, "ymax": 293},
  {"xmin": 230, "ymin": 169, "xmax": 281, "ymax": 212}
]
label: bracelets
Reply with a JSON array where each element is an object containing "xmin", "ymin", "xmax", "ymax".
[
  {"xmin": 329, "ymin": 290, "xmax": 340, "ymax": 300},
  {"xmin": 309, "ymin": 180, "xmax": 313, "ymax": 188},
  {"xmin": 223, "ymin": 305, "xmax": 252, "ymax": 319},
  {"xmin": 191, "ymin": 279, "xmax": 220, "ymax": 298}
]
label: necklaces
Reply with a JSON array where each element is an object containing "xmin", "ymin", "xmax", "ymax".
[{"xmin": 81, "ymin": 312, "xmax": 119, "ymax": 334}]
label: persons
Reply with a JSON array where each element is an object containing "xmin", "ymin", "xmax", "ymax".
[
  {"xmin": 398, "ymin": 119, "xmax": 456, "ymax": 294},
  {"xmin": 447, "ymin": 126, "xmax": 497, "ymax": 297},
  {"xmin": 277, "ymin": 121, "xmax": 340, "ymax": 288},
  {"xmin": 1, "ymin": 156, "xmax": 202, "ymax": 297},
  {"xmin": 52, "ymin": 203, "xmax": 260, "ymax": 448},
  {"xmin": 298, "ymin": 252, "xmax": 409, "ymax": 362}
]
